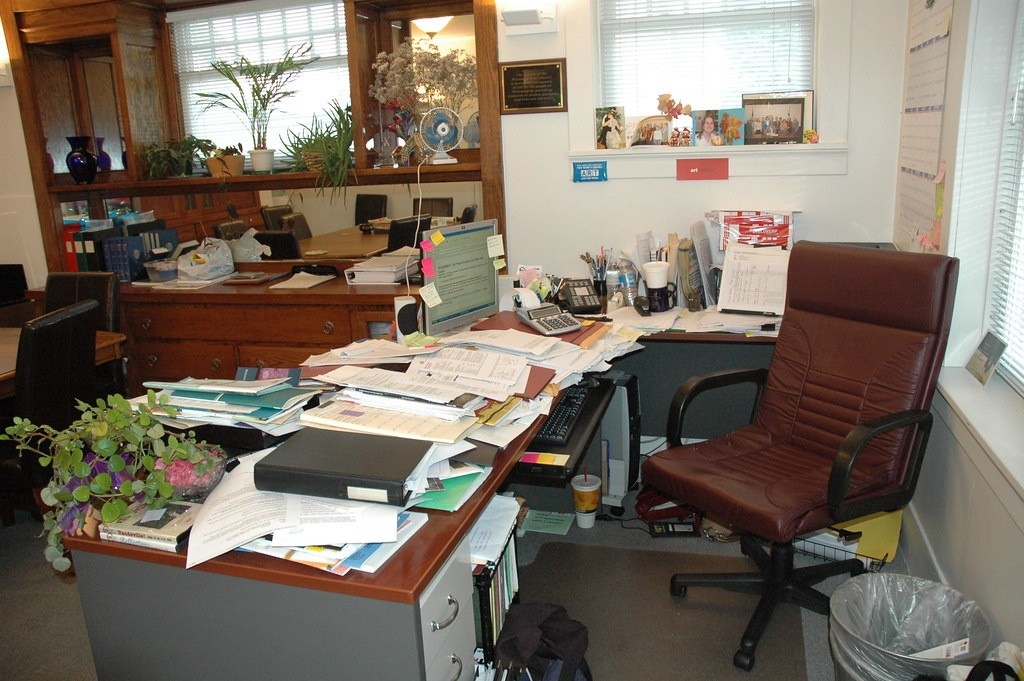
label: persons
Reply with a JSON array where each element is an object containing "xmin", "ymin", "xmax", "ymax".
[
  {"xmin": 681, "ymin": 128, "xmax": 691, "ymax": 146},
  {"xmin": 669, "ymin": 126, "xmax": 680, "ymax": 146},
  {"xmin": 636, "ymin": 122, "xmax": 668, "ymax": 145},
  {"xmin": 745, "ymin": 115, "xmax": 799, "ymax": 144},
  {"xmin": 598, "ymin": 110, "xmax": 623, "ymax": 149},
  {"xmin": 695, "ymin": 112, "xmax": 716, "ymax": 146}
]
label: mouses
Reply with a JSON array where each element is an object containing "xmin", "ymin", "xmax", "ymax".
[{"xmin": 572, "ymin": 375, "xmax": 599, "ymax": 388}]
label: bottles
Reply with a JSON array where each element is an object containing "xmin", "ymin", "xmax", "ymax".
[
  {"xmin": 66, "ymin": 136, "xmax": 98, "ymax": 186},
  {"xmin": 606, "ymin": 261, "xmax": 619, "ymax": 301},
  {"xmin": 619, "ymin": 261, "xmax": 639, "ymax": 306}
]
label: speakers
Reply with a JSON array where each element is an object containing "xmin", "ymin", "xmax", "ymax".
[{"xmin": 393, "ymin": 296, "xmax": 417, "ymax": 340}]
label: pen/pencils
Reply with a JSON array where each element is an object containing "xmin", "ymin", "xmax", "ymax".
[{"xmin": 579, "ymin": 245, "xmax": 613, "ymax": 280}]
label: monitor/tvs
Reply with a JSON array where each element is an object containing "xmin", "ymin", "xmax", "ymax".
[
  {"xmin": 419, "ymin": 219, "xmax": 499, "ymax": 336},
  {"xmin": 388, "ymin": 214, "xmax": 432, "ymax": 253}
]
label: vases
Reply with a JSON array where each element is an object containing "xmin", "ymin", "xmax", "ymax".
[
  {"xmin": 95, "ymin": 137, "xmax": 111, "ymax": 183},
  {"xmin": 412, "ymin": 133, "xmax": 436, "ymax": 165},
  {"xmin": 66, "ymin": 136, "xmax": 98, "ymax": 184},
  {"xmin": 121, "ymin": 137, "xmax": 128, "ymax": 170}
]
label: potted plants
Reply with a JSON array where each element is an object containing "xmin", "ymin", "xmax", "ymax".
[
  {"xmin": 191, "ymin": 40, "xmax": 321, "ymax": 175},
  {"xmin": 0, "ymin": 389, "xmax": 226, "ymax": 575},
  {"xmin": 278, "ymin": 98, "xmax": 359, "ymax": 216},
  {"xmin": 141, "ymin": 133, "xmax": 245, "ymax": 235}
]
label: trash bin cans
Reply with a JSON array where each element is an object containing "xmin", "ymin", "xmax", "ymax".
[{"xmin": 828, "ymin": 573, "xmax": 993, "ymax": 681}]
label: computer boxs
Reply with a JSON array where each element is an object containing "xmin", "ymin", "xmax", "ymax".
[{"xmin": 602, "ymin": 374, "xmax": 642, "ymax": 496}]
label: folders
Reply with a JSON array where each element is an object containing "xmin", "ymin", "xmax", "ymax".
[
  {"xmin": 83, "ymin": 226, "xmax": 125, "ymax": 272},
  {"xmin": 251, "ymin": 426, "xmax": 434, "ymax": 508},
  {"xmin": 140, "ymin": 230, "xmax": 178, "ymax": 256},
  {"xmin": 63, "ymin": 228, "xmax": 81, "ymax": 272},
  {"xmin": 127, "ymin": 219, "xmax": 166, "ymax": 236},
  {"xmin": 73, "ymin": 231, "xmax": 88, "ymax": 271}
]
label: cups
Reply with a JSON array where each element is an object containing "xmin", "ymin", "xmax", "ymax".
[
  {"xmin": 642, "ymin": 261, "xmax": 670, "ymax": 312},
  {"xmin": 571, "ymin": 474, "xmax": 601, "ymax": 529}
]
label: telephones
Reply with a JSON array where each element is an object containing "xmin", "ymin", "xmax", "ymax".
[{"xmin": 553, "ymin": 277, "xmax": 603, "ymax": 313}]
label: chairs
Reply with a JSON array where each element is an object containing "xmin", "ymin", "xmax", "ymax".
[
  {"xmin": 0, "ymin": 263, "xmax": 128, "ymax": 529},
  {"xmin": 461, "ymin": 202, "xmax": 478, "ymax": 225},
  {"xmin": 354, "ymin": 193, "xmax": 387, "ymax": 225},
  {"xmin": 214, "ymin": 203, "xmax": 313, "ymax": 260},
  {"xmin": 388, "ymin": 214, "xmax": 432, "ymax": 252},
  {"xmin": 413, "ymin": 197, "xmax": 453, "ymax": 217},
  {"xmin": 641, "ymin": 240, "xmax": 962, "ymax": 672}
]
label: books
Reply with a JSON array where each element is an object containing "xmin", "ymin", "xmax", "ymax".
[
  {"xmin": 171, "ymin": 238, "xmax": 199, "ymax": 258},
  {"xmin": 487, "ymin": 533, "xmax": 520, "ymax": 645},
  {"xmin": 668, "ymin": 232, "xmax": 701, "ymax": 306},
  {"xmin": 143, "ymin": 247, "xmax": 170, "ymax": 260},
  {"xmin": 440, "ymin": 363, "xmax": 557, "ymax": 427},
  {"xmin": 345, "ymin": 256, "xmax": 418, "ymax": 284},
  {"xmin": 98, "ymin": 375, "xmax": 492, "ymax": 577},
  {"xmin": 122, "ymin": 218, "xmax": 178, "ymax": 258},
  {"xmin": 237, "ymin": 367, "xmax": 325, "ymax": 386},
  {"xmin": 63, "ymin": 225, "xmax": 143, "ymax": 282}
]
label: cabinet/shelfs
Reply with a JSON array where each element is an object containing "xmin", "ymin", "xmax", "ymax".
[
  {"xmin": 22, "ymin": 271, "xmax": 424, "ymax": 400},
  {"xmin": 0, "ymin": 0, "xmax": 510, "ymax": 398}
]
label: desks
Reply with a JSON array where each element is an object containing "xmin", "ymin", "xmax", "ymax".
[
  {"xmin": 63, "ymin": 310, "xmax": 780, "ymax": 681},
  {"xmin": 299, "ymin": 216, "xmax": 462, "ymax": 260},
  {"xmin": 0, "ymin": 325, "xmax": 126, "ymax": 400}
]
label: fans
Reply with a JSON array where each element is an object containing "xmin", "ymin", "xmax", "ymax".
[
  {"xmin": 420, "ymin": 107, "xmax": 464, "ymax": 164},
  {"xmin": 463, "ymin": 112, "xmax": 481, "ymax": 149}
]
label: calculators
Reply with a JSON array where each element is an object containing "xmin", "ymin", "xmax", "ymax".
[{"xmin": 517, "ymin": 302, "xmax": 582, "ymax": 336}]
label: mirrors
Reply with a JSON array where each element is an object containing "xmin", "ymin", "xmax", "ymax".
[
  {"xmin": 58, "ymin": 181, "xmax": 485, "ymax": 263},
  {"xmin": 388, "ymin": 12, "xmax": 481, "ymax": 150},
  {"xmin": 30, "ymin": 36, "xmax": 126, "ymax": 171}
]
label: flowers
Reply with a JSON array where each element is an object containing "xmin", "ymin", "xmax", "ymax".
[
  {"xmin": 428, "ymin": 48, "xmax": 478, "ymax": 115},
  {"xmin": 368, "ymin": 36, "xmax": 453, "ymax": 133},
  {"xmin": 657, "ymin": 93, "xmax": 692, "ymax": 121}
]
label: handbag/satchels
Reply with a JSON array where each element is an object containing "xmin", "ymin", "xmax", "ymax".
[
  {"xmin": 224, "ymin": 228, "xmax": 271, "ymax": 263},
  {"xmin": 492, "ymin": 602, "xmax": 593, "ymax": 681},
  {"xmin": 177, "ymin": 237, "xmax": 234, "ymax": 282}
]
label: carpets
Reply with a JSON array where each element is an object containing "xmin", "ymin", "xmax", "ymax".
[{"xmin": 517, "ymin": 542, "xmax": 808, "ymax": 681}]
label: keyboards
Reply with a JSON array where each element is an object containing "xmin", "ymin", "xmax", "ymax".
[{"xmin": 533, "ymin": 386, "xmax": 590, "ymax": 444}]
